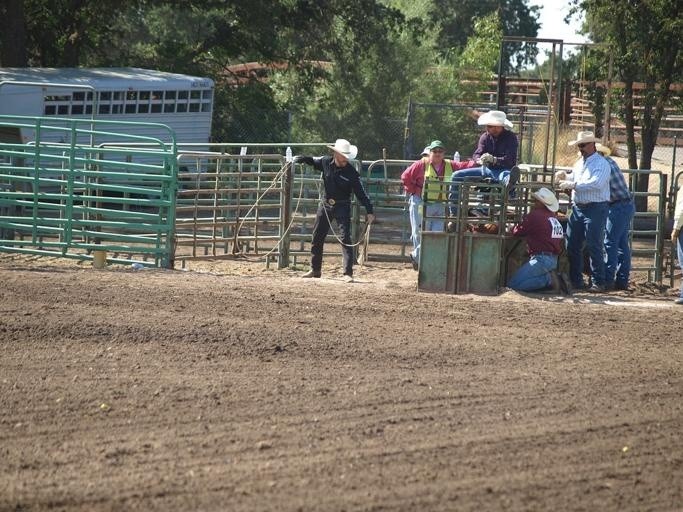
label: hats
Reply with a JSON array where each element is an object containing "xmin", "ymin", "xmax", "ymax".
[
  {"xmin": 420, "ymin": 110, "xmax": 611, "ymax": 212},
  {"xmin": 326, "ymin": 139, "xmax": 357, "ymax": 159}
]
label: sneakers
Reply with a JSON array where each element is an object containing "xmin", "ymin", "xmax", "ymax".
[
  {"xmin": 301, "ymin": 270, "xmax": 320, "ymax": 278},
  {"xmin": 343, "ymin": 274, "xmax": 352, "ymax": 283},
  {"xmin": 550, "ymin": 271, "xmax": 634, "ymax": 295},
  {"xmin": 409, "ymin": 253, "xmax": 417, "ymax": 270}
]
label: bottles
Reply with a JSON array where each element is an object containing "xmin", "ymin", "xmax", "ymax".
[
  {"xmin": 453, "ymin": 151, "xmax": 460, "ymax": 162},
  {"xmin": 130, "ymin": 263, "xmax": 144, "ymax": 270},
  {"xmin": 285, "ymin": 147, "xmax": 292, "ymax": 163}
]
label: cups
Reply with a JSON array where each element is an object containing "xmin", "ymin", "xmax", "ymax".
[{"xmin": 240, "ymin": 147, "xmax": 247, "ymax": 156}]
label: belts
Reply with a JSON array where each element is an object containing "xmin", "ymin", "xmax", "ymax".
[{"xmin": 322, "ymin": 198, "xmax": 347, "ymax": 206}]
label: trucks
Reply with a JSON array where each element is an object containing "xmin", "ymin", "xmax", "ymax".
[{"xmin": 0, "ymin": 67, "xmax": 214, "ymax": 203}]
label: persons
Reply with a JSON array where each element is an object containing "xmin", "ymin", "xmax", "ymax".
[
  {"xmin": 447, "ymin": 110, "xmax": 521, "ymax": 231},
  {"xmin": 289, "ymin": 138, "xmax": 375, "ymax": 283},
  {"xmin": 670, "ymin": 171, "xmax": 682, "ymax": 304},
  {"xmin": 506, "ymin": 188, "xmax": 571, "ymax": 296},
  {"xmin": 403, "ymin": 140, "xmax": 480, "ymax": 271},
  {"xmin": 554, "ymin": 131, "xmax": 635, "ymax": 296}
]
